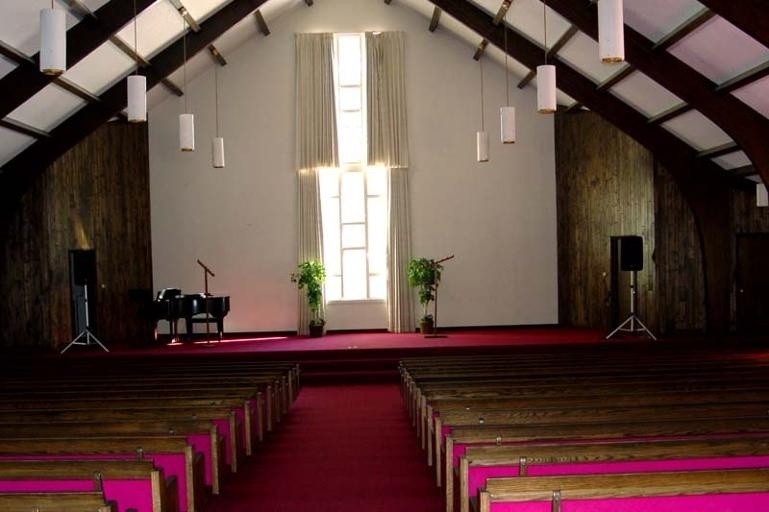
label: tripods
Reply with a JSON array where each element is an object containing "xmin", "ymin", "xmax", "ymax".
[
  {"xmin": 605, "ymin": 279, "xmax": 659, "ymax": 341},
  {"xmin": 60, "ymin": 289, "xmax": 111, "ymax": 356}
]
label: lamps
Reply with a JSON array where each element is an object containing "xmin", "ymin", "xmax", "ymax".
[
  {"xmin": 477, "ymin": 0, "xmax": 624, "ymax": 159},
  {"xmin": 42, "ymin": 0, "xmax": 224, "ymax": 169}
]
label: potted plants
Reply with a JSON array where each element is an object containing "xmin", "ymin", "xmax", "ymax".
[
  {"xmin": 291, "ymin": 260, "xmax": 326, "ymax": 338},
  {"xmin": 407, "ymin": 259, "xmax": 444, "ymax": 332}
]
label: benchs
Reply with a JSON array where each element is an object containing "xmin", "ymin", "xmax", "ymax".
[
  {"xmin": 190, "ymin": 318, "xmax": 222, "ymax": 340},
  {"xmin": 396, "ymin": 343, "xmax": 768, "ymax": 510},
  {"xmin": 2, "ymin": 359, "xmax": 299, "ymax": 510}
]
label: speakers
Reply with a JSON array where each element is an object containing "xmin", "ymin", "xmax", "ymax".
[
  {"xmin": 67, "ymin": 248, "xmax": 98, "ymax": 287},
  {"xmin": 608, "ymin": 234, "xmax": 645, "ymax": 273}
]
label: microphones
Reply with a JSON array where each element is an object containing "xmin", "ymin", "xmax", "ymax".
[{"xmin": 197, "ymin": 258, "xmax": 216, "ymax": 277}]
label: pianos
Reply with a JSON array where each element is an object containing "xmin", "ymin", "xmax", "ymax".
[{"xmin": 153, "ymin": 288, "xmax": 229, "ymax": 344}]
